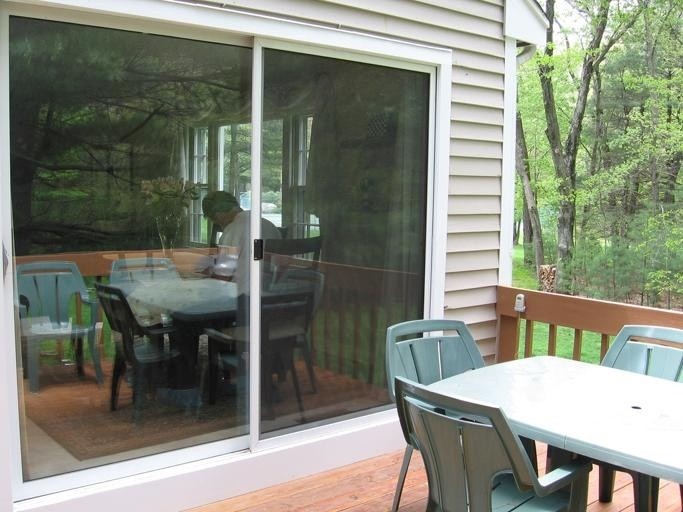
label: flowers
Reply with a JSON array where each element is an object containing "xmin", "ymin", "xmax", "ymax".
[{"xmin": 140, "ymin": 175, "xmax": 201, "ymax": 214}]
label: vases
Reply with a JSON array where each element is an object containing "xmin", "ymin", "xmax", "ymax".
[{"xmin": 154, "ymin": 210, "xmax": 181, "ymax": 258}]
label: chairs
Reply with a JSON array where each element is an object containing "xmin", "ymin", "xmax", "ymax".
[
  {"xmin": 95, "ymin": 284, "xmax": 199, "ymax": 414},
  {"xmin": 273, "ymin": 266, "xmax": 326, "ymax": 392},
  {"xmin": 385, "ymin": 320, "xmax": 486, "ymax": 512},
  {"xmin": 394, "ymin": 376, "xmax": 593, "ymax": 512},
  {"xmin": 14, "ymin": 261, "xmax": 104, "ymax": 393},
  {"xmin": 598, "ymin": 324, "xmax": 683, "ymax": 512},
  {"xmin": 110, "ymin": 258, "xmax": 182, "ymax": 284},
  {"xmin": 205, "ymin": 290, "xmax": 315, "ymax": 423}
]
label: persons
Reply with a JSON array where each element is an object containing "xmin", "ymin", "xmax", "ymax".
[{"xmin": 166, "ymin": 188, "xmax": 283, "ymax": 390}]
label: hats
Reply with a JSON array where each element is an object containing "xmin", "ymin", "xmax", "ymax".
[{"xmin": 202, "ymin": 191, "xmax": 238, "ymax": 215}]
